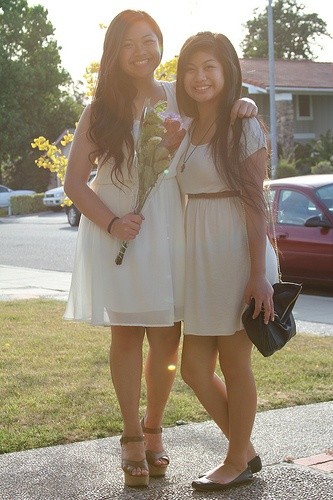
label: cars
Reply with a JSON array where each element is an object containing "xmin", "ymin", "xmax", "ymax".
[
  {"xmin": 263, "ymin": 173, "xmax": 332, "ymax": 287},
  {"xmin": 0, "ymin": 185, "xmax": 37, "ymax": 208},
  {"xmin": 43, "ymin": 171, "xmax": 100, "ymax": 227}
]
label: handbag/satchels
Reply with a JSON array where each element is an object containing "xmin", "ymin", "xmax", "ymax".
[{"xmin": 241, "ymin": 281, "xmax": 303, "ymax": 356}]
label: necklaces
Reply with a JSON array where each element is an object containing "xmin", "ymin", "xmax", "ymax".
[{"xmin": 181, "ymin": 118, "xmax": 216, "ymax": 172}]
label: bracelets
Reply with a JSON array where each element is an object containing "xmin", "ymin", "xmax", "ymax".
[{"xmin": 108, "ymin": 216, "xmax": 119, "ymax": 234}]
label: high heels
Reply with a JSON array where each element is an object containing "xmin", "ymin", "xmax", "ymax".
[
  {"xmin": 140, "ymin": 418, "xmax": 169, "ymax": 476},
  {"xmin": 120, "ymin": 433, "xmax": 149, "ymax": 487}
]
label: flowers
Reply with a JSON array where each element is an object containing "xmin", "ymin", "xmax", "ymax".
[{"xmin": 115, "ymin": 97, "xmax": 187, "ymax": 265}]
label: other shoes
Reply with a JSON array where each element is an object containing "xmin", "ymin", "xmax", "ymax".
[
  {"xmin": 191, "ymin": 467, "xmax": 253, "ymax": 492},
  {"xmin": 197, "ymin": 456, "xmax": 262, "ymax": 479}
]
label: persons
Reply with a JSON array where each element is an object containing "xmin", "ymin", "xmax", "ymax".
[
  {"xmin": 63, "ymin": 9, "xmax": 258, "ymax": 486},
  {"xmin": 177, "ymin": 30, "xmax": 279, "ymax": 490}
]
